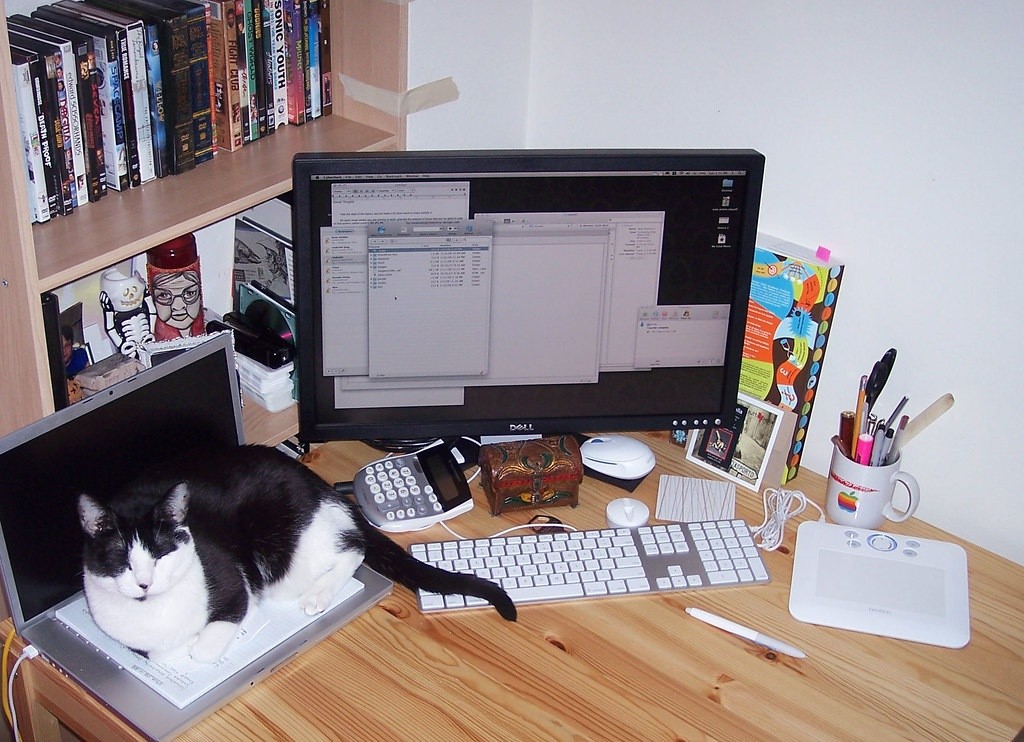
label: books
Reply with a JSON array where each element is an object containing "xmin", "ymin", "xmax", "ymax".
[
  {"xmin": 232, "ymin": 217, "xmax": 293, "ymax": 310},
  {"xmin": 5, "ymin": 0, "xmax": 332, "ymax": 224}
]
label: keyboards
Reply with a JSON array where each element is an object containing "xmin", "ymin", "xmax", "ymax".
[{"xmin": 406, "ymin": 519, "xmax": 769, "ymax": 615}]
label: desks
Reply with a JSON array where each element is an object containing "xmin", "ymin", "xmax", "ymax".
[{"xmin": 0, "ymin": 427, "xmax": 1024, "ymax": 742}]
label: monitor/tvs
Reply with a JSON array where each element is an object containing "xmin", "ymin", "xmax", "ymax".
[{"xmin": 289, "ymin": 148, "xmax": 766, "ymax": 493}]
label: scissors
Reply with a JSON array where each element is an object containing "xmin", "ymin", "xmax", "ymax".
[{"xmin": 865, "ymin": 348, "xmax": 897, "ymax": 415}]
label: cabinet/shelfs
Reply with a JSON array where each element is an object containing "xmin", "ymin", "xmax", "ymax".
[{"xmin": 1, "ymin": 0, "xmax": 409, "ymax": 446}]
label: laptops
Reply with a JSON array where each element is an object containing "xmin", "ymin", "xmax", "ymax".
[{"xmin": 0, "ymin": 334, "xmax": 395, "ymax": 742}]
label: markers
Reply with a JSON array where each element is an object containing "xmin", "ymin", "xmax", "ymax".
[{"xmin": 840, "ymin": 411, "xmax": 874, "ymax": 465}]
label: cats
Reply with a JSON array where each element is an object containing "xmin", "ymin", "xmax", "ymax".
[{"xmin": 77, "ymin": 442, "xmax": 517, "ymax": 663}]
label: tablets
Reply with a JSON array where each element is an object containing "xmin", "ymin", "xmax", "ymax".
[{"xmin": 789, "ymin": 521, "xmax": 971, "ymax": 649}]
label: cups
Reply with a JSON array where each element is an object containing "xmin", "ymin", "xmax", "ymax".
[
  {"xmin": 824, "ymin": 442, "xmax": 920, "ymax": 530},
  {"xmin": 145, "ymin": 231, "xmax": 206, "ymax": 343}
]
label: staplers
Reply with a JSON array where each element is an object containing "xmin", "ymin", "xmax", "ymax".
[{"xmin": 205, "ymin": 311, "xmax": 297, "ymax": 369}]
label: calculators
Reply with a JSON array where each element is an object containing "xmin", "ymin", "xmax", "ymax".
[{"xmin": 354, "ymin": 439, "xmax": 474, "ymax": 532}]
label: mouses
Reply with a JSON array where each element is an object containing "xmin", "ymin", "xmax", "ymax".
[{"xmin": 579, "ymin": 435, "xmax": 656, "ymax": 480}]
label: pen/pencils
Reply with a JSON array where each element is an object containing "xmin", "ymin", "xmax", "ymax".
[
  {"xmin": 885, "ymin": 415, "xmax": 909, "ymax": 465},
  {"xmin": 831, "ymin": 435, "xmax": 852, "ymax": 461},
  {"xmin": 867, "ymin": 396, "xmax": 909, "ymax": 436},
  {"xmin": 878, "ymin": 428, "xmax": 894, "ymax": 466},
  {"xmin": 685, "ymin": 607, "xmax": 810, "ymax": 659},
  {"xmin": 869, "ymin": 424, "xmax": 886, "ymax": 466},
  {"xmin": 851, "ymin": 375, "xmax": 868, "ymax": 458}
]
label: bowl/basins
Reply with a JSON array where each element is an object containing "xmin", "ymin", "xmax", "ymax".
[{"xmin": 606, "ymin": 499, "xmax": 649, "ymax": 528}]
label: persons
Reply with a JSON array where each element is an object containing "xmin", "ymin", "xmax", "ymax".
[
  {"xmin": 88, "ymin": 52, "xmax": 96, "ymax": 68},
  {"xmin": 58, "ymin": 81, "xmax": 64, "ymax": 90},
  {"xmin": 69, "ymin": 173, "xmax": 75, "ymax": 182},
  {"xmin": 285, "ymin": 13, "xmax": 293, "ymax": 34},
  {"xmin": 68, "ymin": 160, "xmax": 74, "ymax": 173},
  {"xmin": 55, "ymin": 55, "xmax": 62, "ymax": 67},
  {"xmin": 325, "ymin": 76, "xmax": 330, "ymax": 104},
  {"xmin": 57, "ymin": 67, "xmax": 63, "ymax": 79},
  {"xmin": 251, "ymin": 97, "xmax": 255, "ymax": 108},
  {"xmin": 67, "ymin": 150, "xmax": 72, "ymax": 159},
  {"xmin": 262, "ymin": 0, "xmax": 269, "ymax": 20},
  {"xmin": 61, "ymin": 325, "xmax": 89, "ymax": 379},
  {"xmin": 238, "ymin": 19, "xmax": 244, "ymax": 33},
  {"xmin": 227, "ymin": 9, "xmax": 235, "ymax": 29},
  {"xmin": 97, "ymin": 150, "xmax": 103, "ymax": 164}
]
